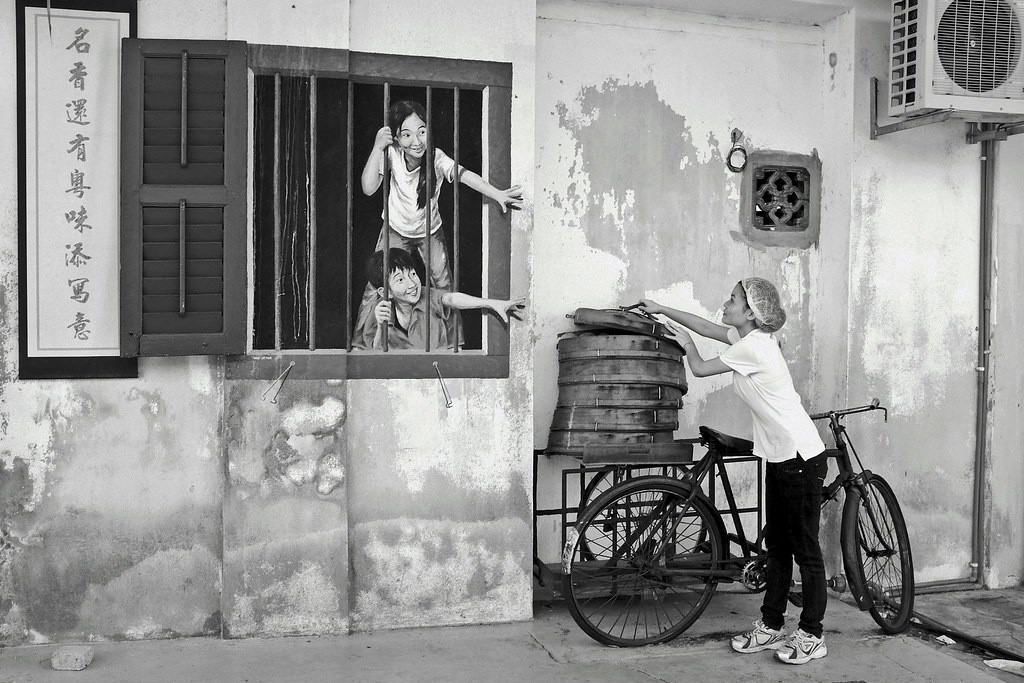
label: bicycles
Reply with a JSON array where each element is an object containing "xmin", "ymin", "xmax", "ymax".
[{"xmin": 558, "ymin": 398, "xmax": 916, "ymax": 651}]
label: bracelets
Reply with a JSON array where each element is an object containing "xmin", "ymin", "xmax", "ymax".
[{"xmin": 683, "ymin": 342, "xmax": 694, "ymax": 349}]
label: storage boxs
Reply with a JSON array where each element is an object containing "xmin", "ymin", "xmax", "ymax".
[{"xmin": 583, "ymin": 441, "xmax": 694, "ymax": 461}]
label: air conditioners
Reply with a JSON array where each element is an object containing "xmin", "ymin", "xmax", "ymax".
[{"xmin": 885, "ymin": 1, "xmax": 1024, "ymax": 124}]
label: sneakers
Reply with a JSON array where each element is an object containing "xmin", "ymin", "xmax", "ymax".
[
  {"xmin": 773, "ymin": 627, "xmax": 828, "ymax": 665},
  {"xmin": 729, "ymin": 619, "xmax": 788, "ymax": 653}
]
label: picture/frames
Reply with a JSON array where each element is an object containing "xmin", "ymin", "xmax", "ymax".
[{"xmin": 16, "ymin": 0, "xmax": 142, "ymax": 382}]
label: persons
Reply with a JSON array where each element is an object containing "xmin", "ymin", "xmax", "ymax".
[{"xmin": 639, "ymin": 278, "xmax": 828, "ymax": 665}]
